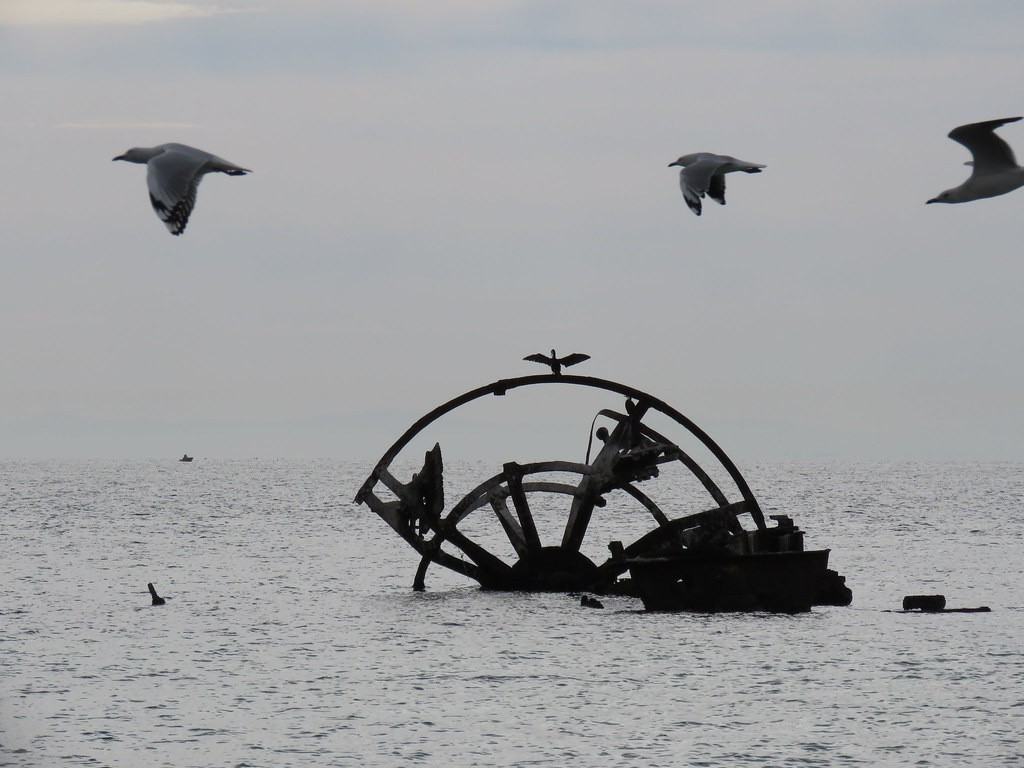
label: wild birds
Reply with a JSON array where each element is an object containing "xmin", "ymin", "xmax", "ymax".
[
  {"xmin": 925, "ymin": 117, "xmax": 1024, "ymax": 205},
  {"xmin": 667, "ymin": 152, "xmax": 767, "ymax": 217},
  {"xmin": 111, "ymin": 142, "xmax": 253, "ymax": 237},
  {"xmin": 522, "ymin": 348, "xmax": 591, "ymax": 376}
]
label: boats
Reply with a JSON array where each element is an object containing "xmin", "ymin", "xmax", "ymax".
[{"xmin": 604, "ymin": 517, "xmax": 833, "ymax": 615}]
